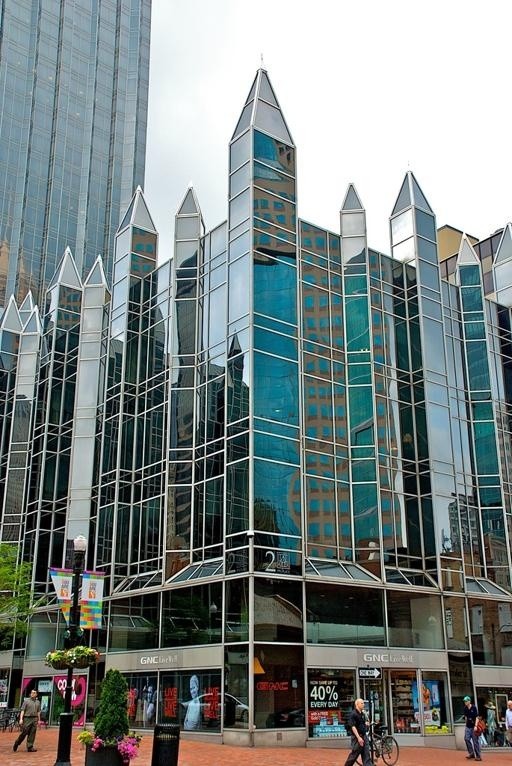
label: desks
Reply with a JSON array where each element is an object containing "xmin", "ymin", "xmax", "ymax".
[{"xmin": 0, "ymin": 709, "xmax": 21, "ymax": 733}]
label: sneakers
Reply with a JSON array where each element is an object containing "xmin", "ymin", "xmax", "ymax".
[
  {"xmin": 13, "ymin": 744, "xmax": 18, "ymax": 751},
  {"xmin": 466, "ymin": 757, "xmax": 481, "ymax": 761},
  {"xmin": 28, "ymin": 748, "xmax": 37, "ymax": 752}
]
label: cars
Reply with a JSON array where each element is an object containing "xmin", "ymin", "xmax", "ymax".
[
  {"xmin": 266, "ymin": 707, "xmax": 305, "ymax": 729},
  {"xmin": 181, "ymin": 692, "xmax": 249, "ymax": 723}
]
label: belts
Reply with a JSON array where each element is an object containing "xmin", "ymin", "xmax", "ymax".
[{"xmin": 25, "ymin": 716, "xmax": 37, "ymax": 719}]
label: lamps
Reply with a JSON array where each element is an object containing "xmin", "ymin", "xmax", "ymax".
[{"xmin": 442, "ymin": 535, "xmax": 452, "ymax": 552}]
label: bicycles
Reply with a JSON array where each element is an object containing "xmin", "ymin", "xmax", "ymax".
[{"xmin": 354, "ymin": 723, "xmax": 399, "ymax": 766}]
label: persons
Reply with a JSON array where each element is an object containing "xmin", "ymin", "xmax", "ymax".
[
  {"xmin": 183, "ymin": 674, "xmax": 203, "ymax": 731},
  {"xmin": 476, "ymin": 699, "xmax": 512, "ymax": 747},
  {"xmin": 13, "ymin": 687, "xmax": 42, "ymax": 753},
  {"xmin": 422, "ymin": 684, "xmax": 431, "ymax": 712},
  {"xmin": 343, "ymin": 698, "xmax": 375, "ymax": 766},
  {"xmin": 124, "ymin": 684, "xmax": 164, "ymax": 726},
  {"xmin": 463, "ymin": 696, "xmax": 482, "ymax": 762}
]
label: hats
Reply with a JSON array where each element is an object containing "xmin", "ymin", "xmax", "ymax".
[
  {"xmin": 485, "ymin": 702, "xmax": 496, "ymax": 710},
  {"xmin": 463, "ymin": 696, "xmax": 471, "ymax": 702}
]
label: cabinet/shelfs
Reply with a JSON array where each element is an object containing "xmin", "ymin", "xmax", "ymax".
[{"xmin": 392, "ymin": 678, "xmax": 415, "ymax": 732}]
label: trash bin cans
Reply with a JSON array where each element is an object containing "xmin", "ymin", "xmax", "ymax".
[
  {"xmin": 151, "ymin": 723, "xmax": 181, "ymax": 766},
  {"xmin": 494, "ymin": 731, "xmax": 504, "ymax": 747}
]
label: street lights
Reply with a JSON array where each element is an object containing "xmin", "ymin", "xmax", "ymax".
[{"xmin": 53, "ymin": 535, "xmax": 88, "ymax": 766}]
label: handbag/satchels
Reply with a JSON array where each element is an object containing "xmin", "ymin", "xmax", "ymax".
[{"xmin": 474, "ymin": 720, "xmax": 484, "ymax": 737}]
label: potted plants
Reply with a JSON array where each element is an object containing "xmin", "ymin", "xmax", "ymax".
[{"xmin": 77, "ymin": 668, "xmax": 143, "ymax": 766}]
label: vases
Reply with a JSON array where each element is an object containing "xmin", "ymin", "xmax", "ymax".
[{"xmin": 51, "ymin": 659, "xmax": 88, "ymax": 670}]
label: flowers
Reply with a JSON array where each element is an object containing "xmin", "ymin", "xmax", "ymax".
[
  {"xmin": 44, "ymin": 646, "xmax": 102, "ymax": 666},
  {"xmin": 77, "ymin": 730, "xmax": 142, "ymax": 763}
]
